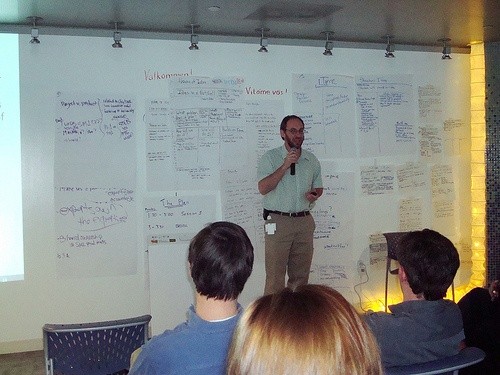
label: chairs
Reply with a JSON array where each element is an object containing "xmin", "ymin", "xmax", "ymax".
[
  {"xmin": 382, "ymin": 346, "xmax": 486, "ymax": 375},
  {"xmin": 382, "ymin": 231, "xmax": 455, "ymax": 313},
  {"xmin": 42, "ymin": 314, "xmax": 154, "ymax": 375}
]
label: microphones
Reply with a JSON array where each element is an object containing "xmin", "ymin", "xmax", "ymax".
[{"xmin": 291, "ymin": 143, "xmax": 297, "ymax": 174}]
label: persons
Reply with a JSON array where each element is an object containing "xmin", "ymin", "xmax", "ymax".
[
  {"xmin": 226, "ymin": 283, "xmax": 384, "ymax": 375},
  {"xmin": 458, "ymin": 280, "xmax": 500, "ymax": 375},
  {"xmin": 363, "ymin": 230, "xmax": 465, "ymax": 375},
  {"xmin": 129, "ymin": 221, "xmax": 254, "ymax": 375},
  {"xmin": 258, "ymin": 115, "xmax": 323, "ymax": 295}
]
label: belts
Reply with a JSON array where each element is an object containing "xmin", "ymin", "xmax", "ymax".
[{"xmin": 268, "ymin": 210, "xmax": 310, "ymax": 217}]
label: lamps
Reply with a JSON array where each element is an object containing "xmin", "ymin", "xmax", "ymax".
[
  {"xmin": 185, "ymin": 24, "xmax": 200, "ymax": 50},
  {"xmin": 254, "ymin": 28, "xmax": 269, "ymax": 53},
  {"xmin": 438, "ymin": 37, "xmax": 453, "ymax": 61},
  {"xmin": 107, "ymin": 21, "xmax": 124, "ymax": 48},
  {"xmin": 381, "ymin": 34, "xmax": 397, "ymax": 59},
  {"xmin": 319, "ymin": 31, "xmax": 334, "ymax": 57},
  {"xmin": 25, "ymin": 16, "xmax": 43, "ymax": 44}
]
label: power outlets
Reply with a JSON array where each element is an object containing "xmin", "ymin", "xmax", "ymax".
[{"xmin": 357, "ymin": 263, "xmax": 366, "ymax": 277}]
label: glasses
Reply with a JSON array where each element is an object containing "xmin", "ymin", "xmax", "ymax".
[{"xmin": 284, "ymin": 127, "xmax": 304, "ymax": 134}]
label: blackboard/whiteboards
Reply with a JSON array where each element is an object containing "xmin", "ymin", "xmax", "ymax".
[{"xmin": 147, "ymin": 239, "xmax": 196, "ymax": 337}]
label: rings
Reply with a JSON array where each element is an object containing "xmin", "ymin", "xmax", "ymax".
[{"xmin": 493, "ymin": 291, "xmax": 496, "ymax": 294}]
